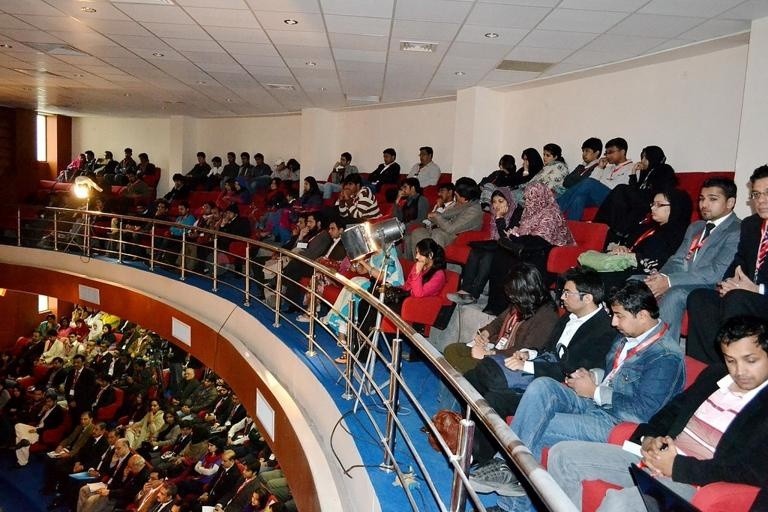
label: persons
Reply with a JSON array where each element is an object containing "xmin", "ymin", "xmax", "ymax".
[
  {"xmin": 685, "ymin": 164, "xmax": 767, "ymax": 367},
  {"xmin": 642, "ymin": 175, "xmax": 742, "ymax": 346},
  {"xmin": 546, "ymin": 313, "xmax": 766, "ymax": 512}
]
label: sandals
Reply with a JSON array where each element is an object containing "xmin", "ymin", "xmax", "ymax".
[
  {"xmin": 337, "ymin": 340, "xmax": 347, "ymax": 347},
  {"xmin": 319, "ymin": 316, "xmax": 328, "ymax": 325}
]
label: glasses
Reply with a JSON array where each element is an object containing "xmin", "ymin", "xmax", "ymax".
[
  {"xmin": 562, "ymin": 289, "xmax": 586, "ymax": 296},
  {"xmin": 650, "ymin": 202, "xmax": 671, "ymax": 208},
  {"xmin": 603, "ymin": 149, "xmax": 619, "ymax": 155},
  {"xmin": 750, "ymin": 190, "xmax": 768, "ymax": 200}
]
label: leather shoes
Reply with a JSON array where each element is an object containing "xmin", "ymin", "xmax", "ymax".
[{"xmin": 282, "ymin": 304, "xmax": 301, "ymax": 313}]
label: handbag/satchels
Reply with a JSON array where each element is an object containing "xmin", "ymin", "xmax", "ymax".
[
  {"xmin": 420, "ymin": 410, "xmax": 463, "ymax": 455},
  {"xmin": 488, "ymin": 342, "xmax": 569, "ymax": 393}
]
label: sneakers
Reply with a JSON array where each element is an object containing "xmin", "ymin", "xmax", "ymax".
[
  {"xmin": 335, "ymin": 353, "xmax": 347, "ymax": 364},
  {"xmin": 469, "ymin": 456, "xmax": 527, "ymax": 496},
  {"xmin": 446, "ymin": 293, "xmax": 477, "ymax": 305},
  {"xmin": 295, "ymin": 312, "xmax": 318, "ymax": 323}
]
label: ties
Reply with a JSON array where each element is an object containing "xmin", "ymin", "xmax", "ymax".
[
  {"xmin": 697, "ymin": 223, "xmax": 715, "ymax": 254},
  {"xmin": 757, "ymin": 224, "xmax": 768, "ymax": 269}
]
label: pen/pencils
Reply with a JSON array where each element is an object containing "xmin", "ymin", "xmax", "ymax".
[
  {"xmin": 477, "ymin": 328, "xmax": 490, "ymax": 348},
  {"xmin": 660, "ymin": 443, "xmax": 668, "ymax": 451},
  {"xmin": 561, "ymin": 369, "xmax": 572, "ymax": 378}
]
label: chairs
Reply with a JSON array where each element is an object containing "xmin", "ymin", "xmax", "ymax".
[{"xmin": 1, "ymin": 164, "xmax": 767, "ymax": 512}]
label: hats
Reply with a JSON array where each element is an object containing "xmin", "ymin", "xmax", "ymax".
[
  {"xmin": 274, "ymin": 159, "xmax": 284, "ymax": 166},
  {"xmin": 225, "ymin": 202, "xmax": 239, "ymax": 214},
  {"xmin": 233, "ymin": 175, "xmax": 246, "ymax": 187}
]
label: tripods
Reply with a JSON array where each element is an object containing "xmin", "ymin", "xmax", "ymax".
[
  {"xmin": 63, "ymin": 209, "xmax": 96, "ymax": 257},
  {"xmin": 333, "ymin": 264, "xmax": 392, "ymax": 415}
]
